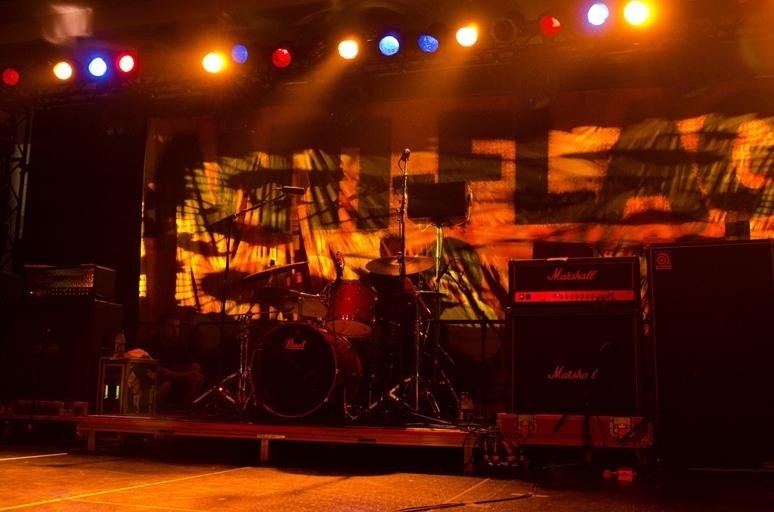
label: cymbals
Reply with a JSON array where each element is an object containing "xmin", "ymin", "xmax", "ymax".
[
  {"xmin": 367, "ymin": 256, "xmax": 435, "ymax": 276},
  {"xmin": 242, "ymin": 261, "xmax": 309, "ymax": 280},
  {"xmin": 395, "ymin": 291, "xmax": 450, "ymax": 298}
]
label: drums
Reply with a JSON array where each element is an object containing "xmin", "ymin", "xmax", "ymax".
[
  {"xmin": 249, "ymin": 321, "xmax": 365, "ymax": 420},
  {"xmin": 323, "ymin": 281, "xmax": 378, "ymax": 338}
]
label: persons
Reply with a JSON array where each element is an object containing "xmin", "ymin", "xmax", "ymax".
[
  {"xmin": 143, "ymin": 317, "xmax": 204, "ymax": 418},
  {"xmin": 362, "ymin": 271, "xmax": 446, "ymax": 403}
]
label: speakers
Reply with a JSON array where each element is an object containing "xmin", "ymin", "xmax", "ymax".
[
  {"xmin": 407, "ymin": 180, "xmax": 472, "ymax": 226},
  {"xmin": 645, "ymin": 237, "xmax": 773, "ymax": 470},
  {"xmin": 504, "ymin": 256, "xmax": 642, "ymax": 416},
  {"xmin": 9, "ymin": 298, "xmax": 123, "ymax": 416}
]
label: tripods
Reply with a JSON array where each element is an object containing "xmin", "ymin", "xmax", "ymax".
[
  {"xmin": 191, "ymin": 195, "xmax": 284, "ymax": 418},
  {"xmin": 351, "ymin": 226, "xmax": 470, "ymax": 426}
]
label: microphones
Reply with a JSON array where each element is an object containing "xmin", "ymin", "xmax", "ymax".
[
  {"xmin": 401, "ymin": 148, "xmax": 410, "ymax": 162},
  {"xmin": 274, "ymin": 185, "xmax": 305, "ymax": 194}
]
